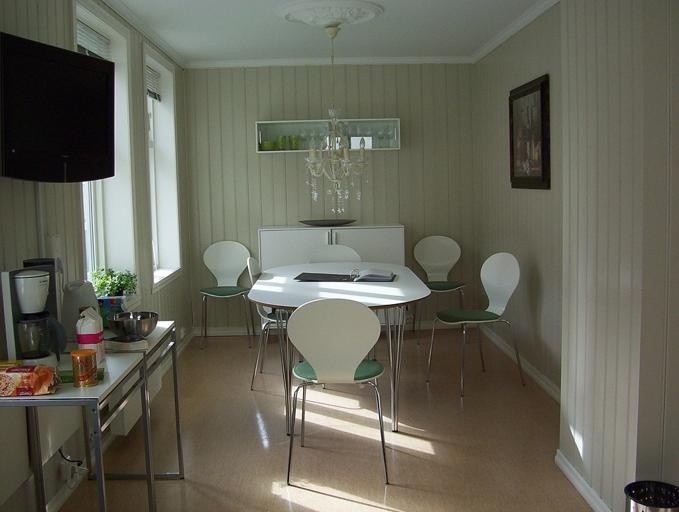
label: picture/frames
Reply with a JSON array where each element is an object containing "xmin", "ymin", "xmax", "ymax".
[{"xmin": 508, "ymin": 74, "xmax": 551, "ymax": 190}]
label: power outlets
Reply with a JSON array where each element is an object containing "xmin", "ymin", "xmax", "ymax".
[{"xmin": 60, "ymin": 455, "xmax": 77, "ymax": 479}]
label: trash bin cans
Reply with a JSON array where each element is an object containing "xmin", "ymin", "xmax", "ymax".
[{"xmin": 624, "ymin": 480, "xmax": 679, "ymax": 512}]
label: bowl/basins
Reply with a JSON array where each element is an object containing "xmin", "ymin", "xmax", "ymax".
[{"xmin": 107, "ymin": 311, "xmax": 158, "ymax": 341}]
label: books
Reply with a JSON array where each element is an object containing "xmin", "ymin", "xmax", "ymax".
[{"xmin": 293, "ymin": 272, "xmax": 397, "ymax": 282}]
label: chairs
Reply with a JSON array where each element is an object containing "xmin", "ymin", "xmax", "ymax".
[
  {"xmin": 425, "ymin": 252, "xmax": 526, "ymax": 396},
  {"xmin": 286, "ymin": 297, "xmax": 390, "ymax": 485},
  {"xmin": 412, "ymin": 235, "xmax": 467, "ymax": 344},
  {"xmin": 246, "ymin": 257, "xmax": 290, "ymax": 436},
  {"xmin": 199, "ymin": 240, "xmax": 254, "ymax": 350},
  {"xmin": 309, "ymin": 245, "xmax": 361, "ymax": 263}
]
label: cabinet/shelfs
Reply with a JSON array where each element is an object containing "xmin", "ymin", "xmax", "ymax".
[{"xmin": 258, "ymin": 230, "xmax": 403, "ymax": 326}]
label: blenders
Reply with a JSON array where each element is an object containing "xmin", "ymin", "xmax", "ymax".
[{"xmin": 0, "ymin": 266, "xmax": 60, "ymax": 370}]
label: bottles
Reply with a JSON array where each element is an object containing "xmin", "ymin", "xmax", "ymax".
[{"xmin": 355, "ymin": 124, "xmax": 395, "ymax": 149}]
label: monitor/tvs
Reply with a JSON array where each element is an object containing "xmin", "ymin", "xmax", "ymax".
[{"xmin": 0, "ymin": 30, "xmax": 115, "ymax": 183}]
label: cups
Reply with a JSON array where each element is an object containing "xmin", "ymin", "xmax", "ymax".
[{"xmin": 260, "ymin": 133, "xmax": 301, "ymax": 150}]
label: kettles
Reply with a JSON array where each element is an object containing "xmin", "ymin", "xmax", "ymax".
[{"xmin": 62, "ymin": 278, "xmax": 102, "ymax": 344}]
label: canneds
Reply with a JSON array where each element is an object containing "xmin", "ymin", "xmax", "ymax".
[{"xmin": 71, "ymin": 349, "xmax": 98, "ymax": 386}]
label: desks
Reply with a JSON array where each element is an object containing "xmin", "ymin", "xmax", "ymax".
[{"xmin": 0, "ymin": 320, "xmax": 184, "ymax": 512}]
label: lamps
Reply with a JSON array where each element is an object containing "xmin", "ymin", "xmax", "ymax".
[{"xmin": 284, "ymin": 2, "xmax": 382, "ymax": 214}]
